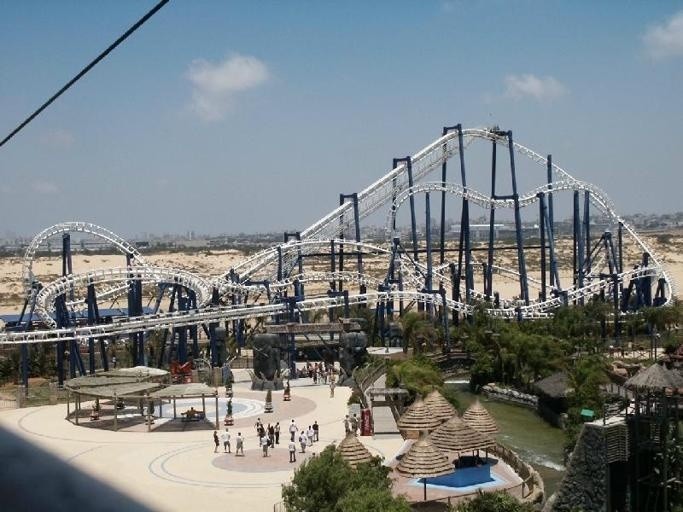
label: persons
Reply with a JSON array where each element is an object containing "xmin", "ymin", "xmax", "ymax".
[
  {"xmin": 341, "ymin": 413, "xmax": 350, "ymax": 435},
  {"xmin": 211, "ymin": 416, "xmax": 319, "ymax": 463},
  {"xmin": 187, "ymin": 407, "xmax": 195, "ymax": 418},
  {"xmin": 348, "ymin": 412, "xmax": 359, "ymax": 437},
  {"xmin": 295, "ymin": 360, "xmax": 339, "ymax": 397}
]
